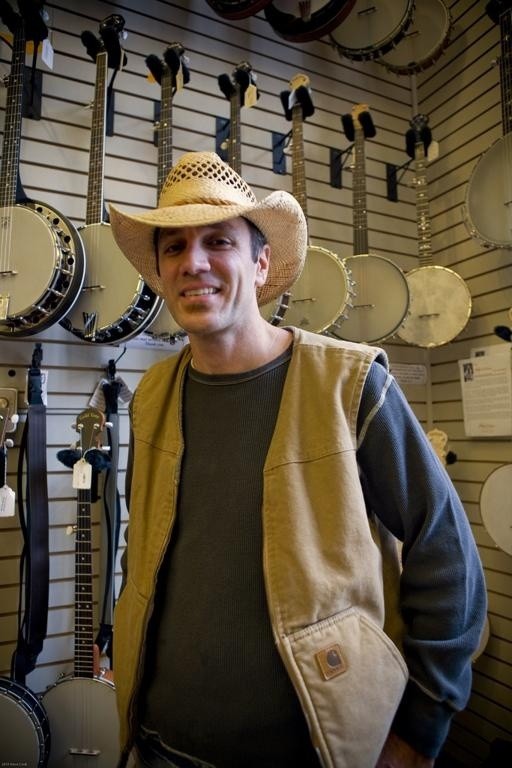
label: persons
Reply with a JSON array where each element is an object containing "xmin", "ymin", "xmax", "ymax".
[{"xmin": 108, "ymin": 151, "xmax": 488, "ymax": 768}]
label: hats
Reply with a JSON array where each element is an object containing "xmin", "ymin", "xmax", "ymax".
[{"xmin": 106, "ymin": 150, "xmax": 309, "ymax": 310}]
